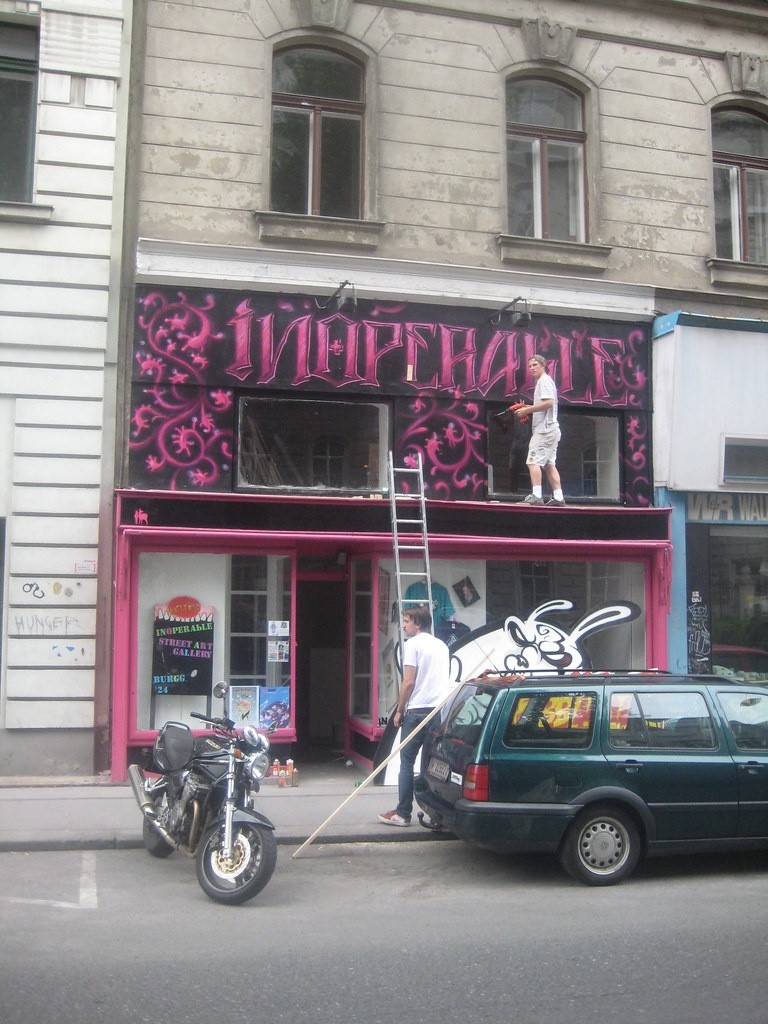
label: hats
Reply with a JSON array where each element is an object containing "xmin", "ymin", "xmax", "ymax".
[{"xmin": 529, "ymin": 355, "xmax": 550, "ymax": 374}]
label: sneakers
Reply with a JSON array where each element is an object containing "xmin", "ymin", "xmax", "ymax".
[
  {"xmin": 378, "ymin": 809, "xmax": 411, "ymax": 827},
  {"xmin": 515, "ymin": 493, "xmax": 545, "ymax": 506},
  {"xmin": 545, "ymin": 498, "xmax": 565, "ymax": 507}
]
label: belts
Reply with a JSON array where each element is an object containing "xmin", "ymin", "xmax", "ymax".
[{"xmin": 407, "ymin": 708, "xmax": 441, "ymax": 715}]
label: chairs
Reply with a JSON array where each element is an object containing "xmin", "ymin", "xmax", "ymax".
[{"xmin": 674, "ymin": 717, "xmax": 707, "ymax": 748}]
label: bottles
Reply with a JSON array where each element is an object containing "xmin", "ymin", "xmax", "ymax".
[
  {"xmin": 268, "ymin": 759, "xmax": 299, "ymax": 788},
  {"xmin": 355, "ymin": 781, "xmax": 362, "ymax": 787}
]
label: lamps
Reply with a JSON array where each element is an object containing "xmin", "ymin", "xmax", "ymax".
[
  {"xmin": 315, "ymin": 281, "xmax": 358, "ymax": 312},
  {"xmin": 491, "ymin": 296, "xmax": 532, "ymax": 326}
]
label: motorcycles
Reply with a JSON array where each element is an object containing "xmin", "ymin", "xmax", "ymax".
[{"xmin": 127, "ymin": 681, "xmax": 278, "ymax": 905}]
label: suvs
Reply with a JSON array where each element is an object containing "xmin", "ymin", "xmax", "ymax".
[{"xmin": 413, "ymin": 668, "xmax": 768, "ymax": 886}]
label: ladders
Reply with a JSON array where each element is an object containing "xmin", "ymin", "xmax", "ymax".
[{"xmin": 386, "ymin": 449, "xmax": 435, "ymax": 680}]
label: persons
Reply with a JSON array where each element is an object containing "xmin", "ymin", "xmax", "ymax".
[
  {"xmin": 462, "ymin": 586, "xmax": 480, "ymax": 607},
  {"xmin": 378, "ymin": 606, "xmax": 450, "ymax": 826},
  {"xmin": 515, "ymin": 355, "xmax": 567, "ymax": 506}
]
